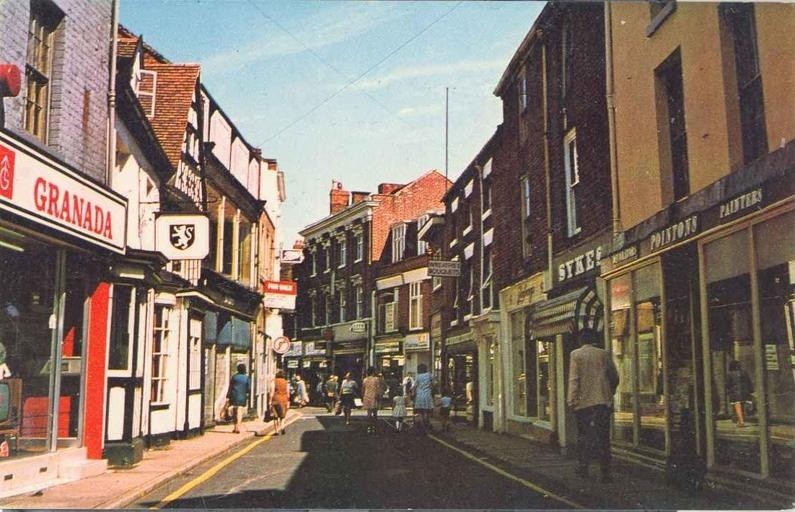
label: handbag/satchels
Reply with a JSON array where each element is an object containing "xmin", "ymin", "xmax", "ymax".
[
  {"xmin": 264, "ymin": 406, "xmax": 279, "ymax": 423},
  {"xmin": 218, "ymin": 401, "xmax": 234, "ymax": 422}
]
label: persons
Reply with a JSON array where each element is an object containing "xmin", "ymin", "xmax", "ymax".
[
  {"xmin": 288, "ymin": 363, "xmax": 453, "ymax": 438},
  {"xmin": 725, "ymin": 356, "xmax": 755, "ymax": 427},
  {"xmin": 566, "ymin": 326, "xmax": 622, "ymax": 481},
  {"xmin": 268, "ymin": 370, "xmax": 290, "ymax": 436},
  {"xmin": 226, "ymin": 363, "xmax": 251, "ymax": 433}
]
